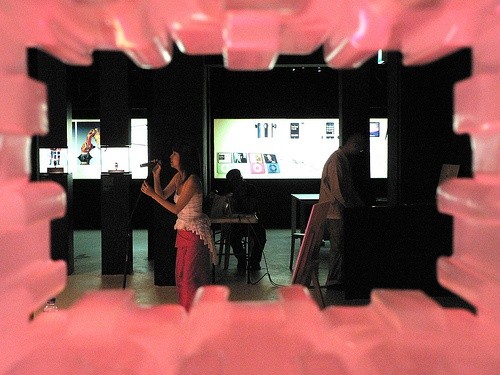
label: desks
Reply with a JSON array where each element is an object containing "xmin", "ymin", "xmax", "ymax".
[
  {"xmin": 209, "ymin": 212, "xmax": 259, "ymax": 284},
  {"xmin": 290, "ymin": 193, "xmax": 320, "ymax": 271}
]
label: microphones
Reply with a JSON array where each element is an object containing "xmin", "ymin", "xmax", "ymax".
[{"xmin": 140, "ymin": 159, "xmax": 162, "ymax": 167}]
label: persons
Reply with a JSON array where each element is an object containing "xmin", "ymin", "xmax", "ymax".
[
  {"xmin": 235, "ymin": 153, "xmax": 247, "ymax": 163},
  {"xmin": 141, "ymin": 138, "xmax": 217, "ymax": 313},
  {"xmin": 319, "ymin": 131, "xmax": 372, "ymax": 292},
  {"xmin": 216, "ymin": 169, "xmax": 266, "ymax": 274},
  {"xmin": 266, "ymin": 155, "xmax": 274, "ymax": 162}
]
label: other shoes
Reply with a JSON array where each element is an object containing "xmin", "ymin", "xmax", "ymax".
[
  {"xmin": 237, "ymin": 260, "xmax": 246, "ymax": 270},
  {"xmin": 249, "ymin": 262, "xmax": 261, "ymax": 270}
]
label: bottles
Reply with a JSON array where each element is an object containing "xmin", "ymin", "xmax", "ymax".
[
  {"xmin": 42, "ymin": 298, "xmax": 59, "ymax": 313},
  {"xmin": 115, "ymin": 163, "xmax": 118, "ymax": 170},
  {"xmin": 251, "ymin": 212, "xmax": 258, "ymax": 223}
]
